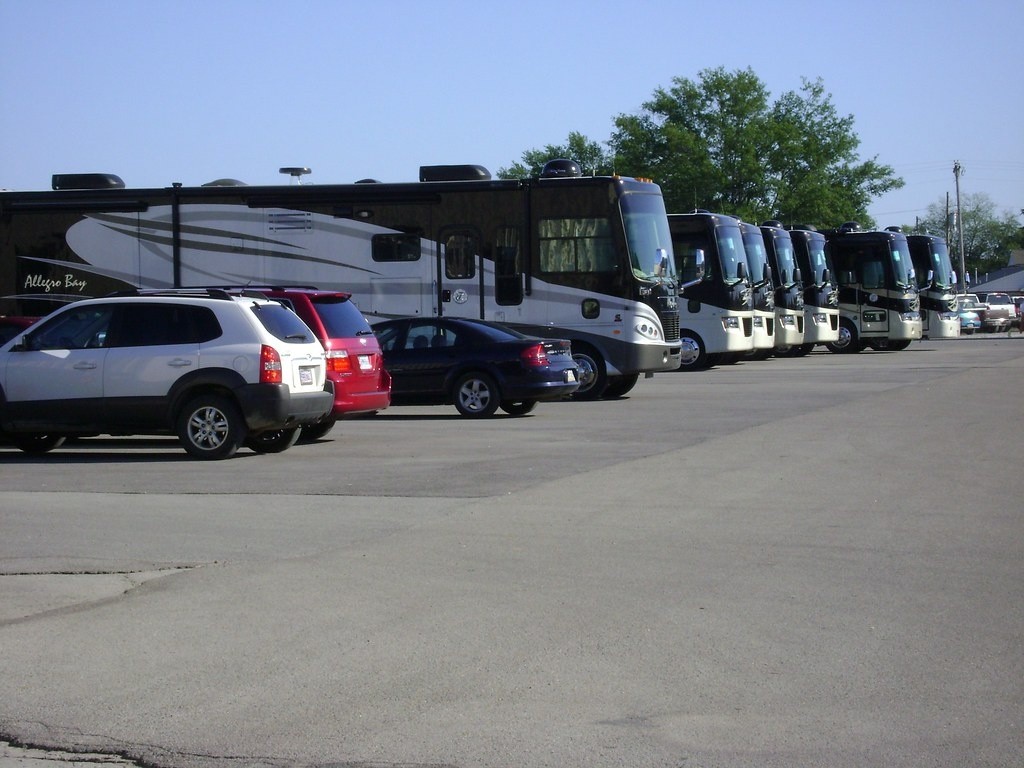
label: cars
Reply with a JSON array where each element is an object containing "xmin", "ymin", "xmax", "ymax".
[
  {"xmin": 368, "ymin": 315, "xmax": 581, "ymax": 419},
  {"xmin": 956, "ymin": 300, "xmax": 981, "ymax": 335},
  {"xmin": 979, "ymin": 292, "xmax": 1024, "ymax": 332}
]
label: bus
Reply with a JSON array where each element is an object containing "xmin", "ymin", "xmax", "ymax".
[
  {"xmin": 664, "ymin": 208, "xmax": 972, "ymax": 371},
  {"xmin": 0, "ymin": 161, "xmax": 707, "ymax": 401}
]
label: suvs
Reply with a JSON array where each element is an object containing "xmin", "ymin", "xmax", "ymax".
[
  {"xmin": 0, "ymin": 285, "xmax": 335, "ymax": 460},
  {"xmin": 0, "ymin": 282, "xmax": 394, "ymax": 447}
]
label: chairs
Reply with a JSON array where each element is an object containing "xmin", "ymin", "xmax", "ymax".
[{"xmin": 413, "ymin": 334, "xmax": 447, "ymax": 348}]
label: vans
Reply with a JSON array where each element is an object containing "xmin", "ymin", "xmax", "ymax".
[{"xmin": 956, "ymin": 294, "xmax": 982, "ymax": 318}]
